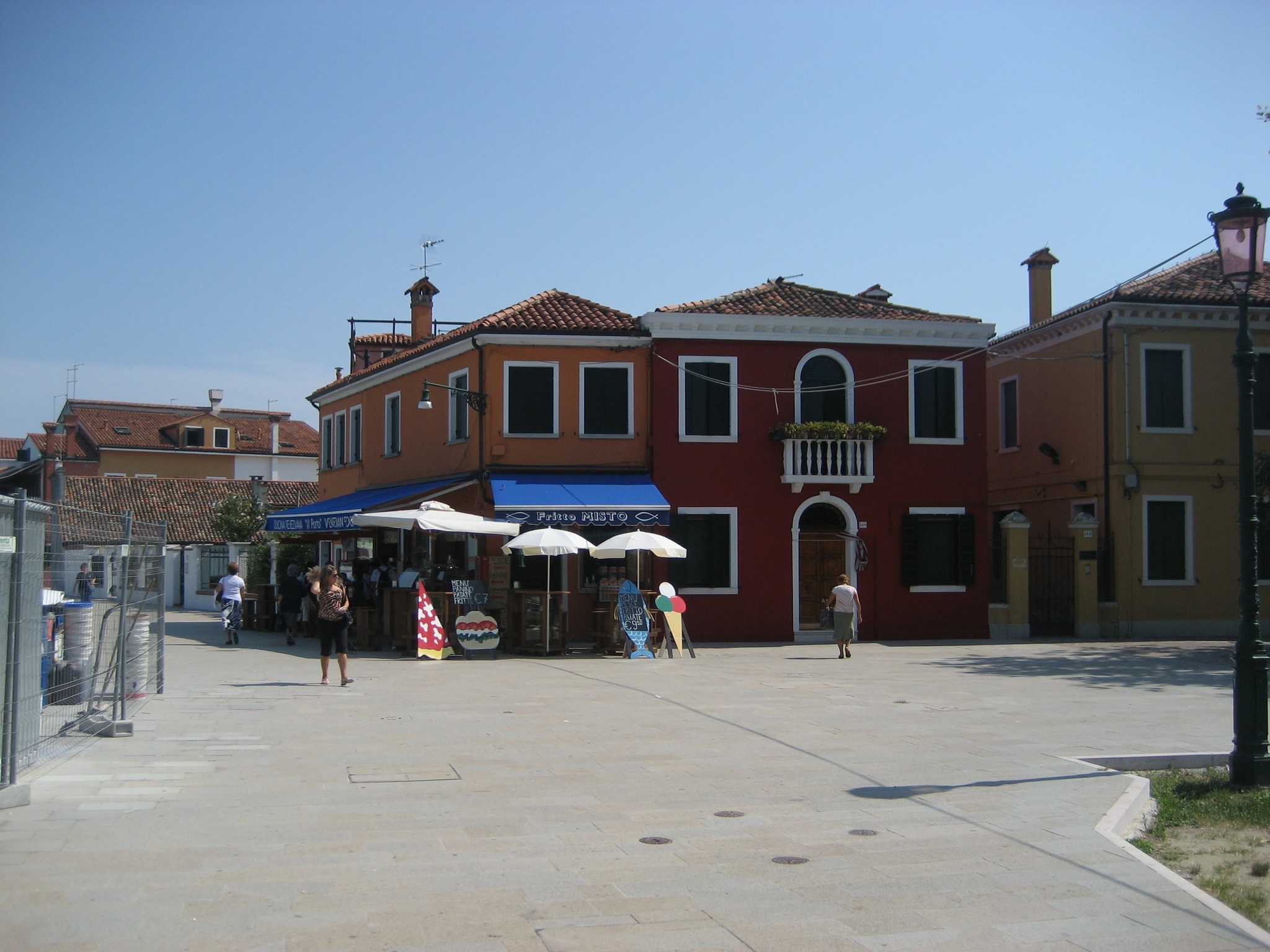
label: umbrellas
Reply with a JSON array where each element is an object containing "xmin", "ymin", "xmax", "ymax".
[
  {"xmin": 591, "ymin": 528, "xmax": 687, "ymax": 590},
  {"xmin": 501, "ymin": 526, "xmax": 596, "ymax": 652},
  {"xmin": 350, "ymin": 500, "xmax": 520, "ymax": 562}
]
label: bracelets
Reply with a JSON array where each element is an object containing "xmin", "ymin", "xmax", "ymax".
[
  {"xmin": 278, "ymin": 602, "xmax": 280, "ymax": 604},
  {"xmin": 344, "ymin": 606, "xmax": 347, "ymax": 609}
]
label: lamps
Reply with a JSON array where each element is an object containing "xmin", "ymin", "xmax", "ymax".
[
  {"xmin": 418, "ymin": 379, "xmax": 487, "ymax": 415},
  {"xmin": 1074, "ymin": 480, "xmax": 1087, "ymax": 492},
  {"xmin": 1039, "ymin": 442, "xmax": 1059, "ymax": 465}
]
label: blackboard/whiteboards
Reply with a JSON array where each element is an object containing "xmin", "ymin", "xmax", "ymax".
[
  {"xmin": 619, "ymin": 593, "xmax": 648, "ymax": 631},
  {"xmin": 452, "ymin": 579, "xmax": 489, "ymax": 604}
]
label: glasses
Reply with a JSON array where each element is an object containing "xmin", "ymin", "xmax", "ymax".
[{"xmin": 331, "ymin": 573, "xmax": 338, "ymax": 578}]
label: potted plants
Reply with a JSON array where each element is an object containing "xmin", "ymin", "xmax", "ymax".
[{"xmin": 779, "ymin": 420, "xmax": 887, "ymax": 441}]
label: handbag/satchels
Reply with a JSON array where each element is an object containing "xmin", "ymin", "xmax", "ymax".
[
  {"xmin": 820, "ymin": 606, "xmax": 834, "ymax": 630},
  {"xmin": 215, "ymin": 589, "xmax": 223, "ymax": 603},
  {"xmin": 89, "ymin": 584, "xmax": 95, "ymax": 592},
  {"xmin": 340, "ymin": 602, "xmax": 355, "ymax": 627}
]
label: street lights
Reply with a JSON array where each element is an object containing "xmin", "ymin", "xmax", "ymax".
[{"xmin": 1207, "ymin": 178, "xmax": 1270, "ymax": 782}]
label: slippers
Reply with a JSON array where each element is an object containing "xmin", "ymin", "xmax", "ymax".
[
  {"xmin": 322, "ymin": 679, "xmax": 330, "ymax": 685},
  {"xmin": 341, "ymin": 678, "xmax": 354, "ymax": 686}
]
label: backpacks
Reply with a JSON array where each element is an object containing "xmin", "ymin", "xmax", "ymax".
[{"xmin": 375, "ymin": 565, "xmax": 393, "ymax": 597}]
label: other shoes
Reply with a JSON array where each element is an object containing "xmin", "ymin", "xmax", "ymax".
[
  {"xmin": 225, "ymin": 640, "xmax": 233, "ymax": 644},
  {"xmin": 839, "ymin": 654, "xmax": 844, "ymax": 659},
  {"xmin": 287, "ymin": 634, "xmax": 295, "ymax": 645},
  {"xmin": 303, "ymin": 633, "xmax": 314, "ymax": 638},
  {"xmin": 233, "ymin": 631, "xmax": 239, "ymax": 644},
  {"xmin": 845, "ymin": 647, "xmax": 851, "ymax": 658}
]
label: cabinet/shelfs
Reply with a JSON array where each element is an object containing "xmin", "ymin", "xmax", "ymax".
[
  {"xmin": 255, "ymin": 584, "xmax": 279, "ymax": 632},
  {"xmin": 240, "ymin": 598, "xmax": 258, "ymax": 630},
  {"xmin": 352, "ymin": 606, "xmax": 382, "ymax": 651},
  {"xmin": 373, "ymin": 587, "xmax": 418, "ymax": 652},
  {"xmin": 591, "ymin": 590, "xmax": 662, "ymax": 655},
  {"xmin": 402, "ymin": 590, "xmax": 460, "ymax": 660},
  {"xmin": 514, "ymin": 590, "xmax": 570, "ymax": 657}
]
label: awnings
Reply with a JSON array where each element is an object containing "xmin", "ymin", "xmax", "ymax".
[
  {"xmin": 486, "ymin": 464, "xmax": 672, "ymax": 526},
  {"xmin": 258, "ymin": 470, "xmax": 479, "ymax": 535}
]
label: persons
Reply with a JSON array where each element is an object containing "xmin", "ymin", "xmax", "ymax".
[
  {"xmin": 277, "ymin": 565, "xmax": 317, "ymax": 648},
  {"xmin": 307, "ymin": 564, "xmax": 354, "ymax": 687},
  {"xmin": 825, "ymin": 574, "xmax": 862, "ymax": 659},
  {"xmin": 214, "ymin": 561, "xmax": 246, "ymax": 646},
  {"xmin": 72, "ymin": 563, "xmax": 96, "ymax": 603},
  {"xmin": 338, "ymin": 551, "xmax": 434, "ymax": 651}
]
